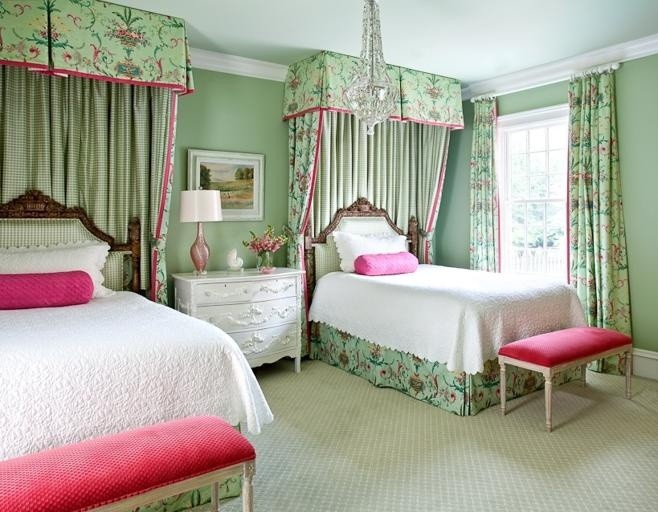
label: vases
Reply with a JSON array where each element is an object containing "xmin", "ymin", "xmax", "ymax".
[{"xmin": 254, "ymin": 251, "xmax": 274, "ymax": 272}]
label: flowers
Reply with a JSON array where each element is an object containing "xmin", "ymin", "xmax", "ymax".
[{"xmin": 238, "ymin": 221, "xmax": 289, "ymax": 268}]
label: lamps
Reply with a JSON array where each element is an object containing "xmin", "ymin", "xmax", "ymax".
[
  {"xmin": 178, "ymin": 187, "xmax": 224, "ymax": 274},
  {"xmin": 343, "ymin": 2, "xmax": 398, "ymax": 139}
]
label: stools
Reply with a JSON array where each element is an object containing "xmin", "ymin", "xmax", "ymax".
[
  {"xmin": 2, "ymin": 413, "xmax": 260, "ymax": 512},
  {"xmin": 495, "ymin": 324, "xmax": 633, "ymax": 431}
]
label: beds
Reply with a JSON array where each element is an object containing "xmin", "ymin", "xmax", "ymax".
[
  {"xmin": 300, "ymin": 197, "xmax": 589, "ymax": 418},
  {"xmin": 1, "ymin": 183, "xmax": 276, "ymax": 511}
]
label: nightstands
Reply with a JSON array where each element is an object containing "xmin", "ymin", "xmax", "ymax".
[{"xmin": 169, "ymin": 266, "xmax": 308, "ymax": 376}]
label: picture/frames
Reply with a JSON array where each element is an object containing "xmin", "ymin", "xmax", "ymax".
[{"xmin": 184, "ymin": 148, "xmax": 267, "ymax": 223}]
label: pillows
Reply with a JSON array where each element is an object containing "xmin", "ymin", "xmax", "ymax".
[
  {"xmin": 1, "ymin": 269, "xmax": 94, "ymax": 309},
  {"xmin": 332, "ymin": 227, "xmax": 407, "ymax": 273},
  {"xmin": 1, "ymin": 239, "xmax": 116, "ymax": 299},
  {"xmin": 353, "ymin": 250, "xmax": 419, "ymax": 275}
]
